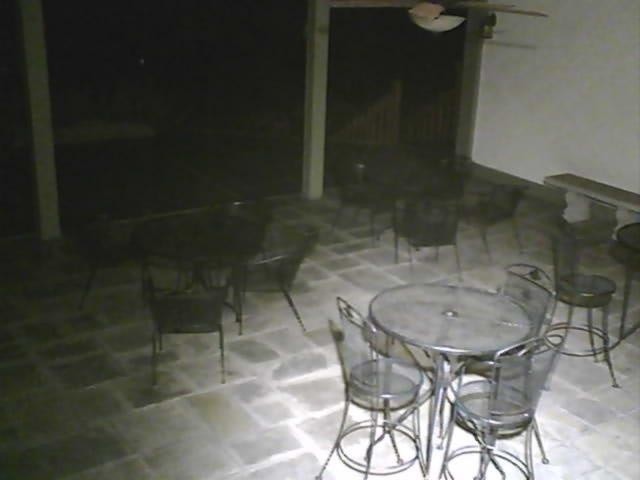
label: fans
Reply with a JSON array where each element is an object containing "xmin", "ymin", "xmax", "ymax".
[{"xmin": 325, "ymin": 0, "xmax": 553, "ymax": 25}]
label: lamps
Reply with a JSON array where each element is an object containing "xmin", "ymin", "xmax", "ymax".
[
  {"xmin": 409, "ymin": 5, "xmax": 467, "ymax": 35},
  {"xmin": 480, "ymin": 12, "xmax": 497, "ymax": 40}
]
